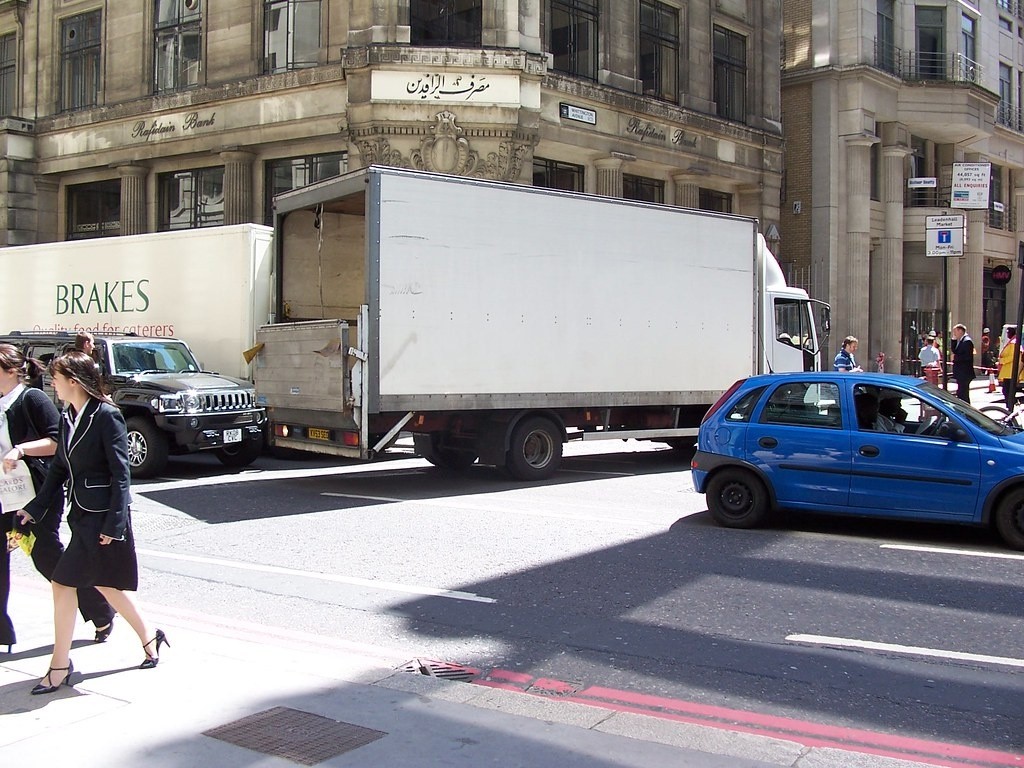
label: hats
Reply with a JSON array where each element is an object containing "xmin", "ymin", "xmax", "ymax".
[
  {"xmin": 878, "ymin": 389, "xmax": 911, "ymax": 402},
  {"xmin": 929, "ymin": 330, "xmax": 936, "ymax": 337},
  {"xmin": 984, "ymin": 328, "xmax": 990, "ymax": 333},
  {"xmin": 926, "ymin": 337, "xmax": 935, "ymax": 340}
]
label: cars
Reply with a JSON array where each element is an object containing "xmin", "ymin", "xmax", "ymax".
[{"xmin": 690, "ymin": 371, "xmax": 1024, "ymax": 552}]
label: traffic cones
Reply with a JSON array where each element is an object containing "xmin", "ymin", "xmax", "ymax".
[{"xmin": 985, "ymin": 369, "xmax": 1000, "ymax": 393}]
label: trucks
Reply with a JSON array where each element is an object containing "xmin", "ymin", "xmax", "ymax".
[{"xmin": 252, "ymin": 164, "xmax": 832, "ymax": 482}]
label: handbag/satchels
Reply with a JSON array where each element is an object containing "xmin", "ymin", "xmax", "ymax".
[{"xmin": 25, "ymin": 456, "xmax": 69, "ymax": 493}]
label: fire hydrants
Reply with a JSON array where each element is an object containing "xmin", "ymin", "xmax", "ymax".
[
  {"xmin": 924, "ymin": 361, "xmax": 943, "ymax": 416},
  {"xmin": 875, "ymin": 352, "xmax": 886, "ymax": 373}
]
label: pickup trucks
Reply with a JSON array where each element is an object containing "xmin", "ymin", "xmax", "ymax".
[{"xmin": 0, "ymin": 331, "xmax": 268, "ymax": 477}]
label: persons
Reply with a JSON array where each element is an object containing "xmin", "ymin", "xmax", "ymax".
[
  {"xmin": 73, "ymin": 333, "xmax": 96, "ymax": 354},
  {"xmin": 0, "ymin": 343, "xmax": 117, "ymax": 652},
  {"xmin": 917, "ymin": 331, "xmax": 943, "ymax": 387},
  {"xmin": 950, "ymin": 324, "xmax": 975, "ymax": 404},
  {"xmin": 990, "ymin": 327, "xmax": 1024, "ymax": 420},
  {"xmin": 830, "ymin": 335, "xmax": 863, "ymax": 404},
  {"xmin": 981, "ymin": 328, "xmax": 990, "ymax": 376},
  {"xmin": 16, "ymin": 351, "xmax": 171, "ymax": 694},
  {"xmin": 854, "ymin": 385, "xmax": 908, "ymax": 433}
]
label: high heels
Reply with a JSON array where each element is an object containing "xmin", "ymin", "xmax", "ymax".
[
  {"xmin": 31, "ymin": 658, "xmax": 74, "ymax": 694},
  {"xmin": 0, "ymin": 642, "xmax": 15, "ymax": 654},
  {"xmin": 140, "ymin": 628, "xmax": 170, "ymax": 668},
  {"xmin": 95, "ymin": 620, "xmax": 114, "ymax": 643}
]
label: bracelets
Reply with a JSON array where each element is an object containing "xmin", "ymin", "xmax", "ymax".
[{"xmin": 14, "ymin": 444, "xmax": 26, "ymax": 458}]
